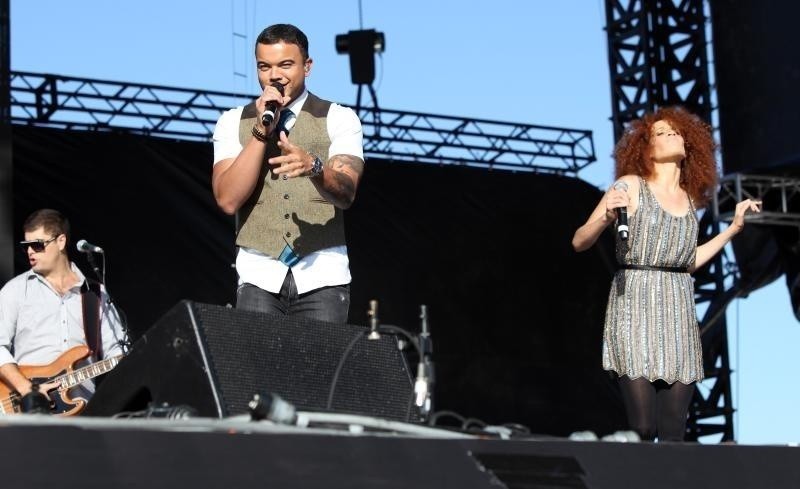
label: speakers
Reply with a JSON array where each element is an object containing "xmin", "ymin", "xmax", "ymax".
[{"xmin": 79, "ymin": 301, "xmax": 420, "ymax": 424}]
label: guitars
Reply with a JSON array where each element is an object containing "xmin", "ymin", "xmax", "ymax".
[{"xmin": 0, "ymin": 346, "xmax": 130, "ymax": 416}]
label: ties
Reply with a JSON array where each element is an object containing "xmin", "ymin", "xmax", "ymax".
[{"xmin": 278, "ymin": 108, "xmax": 301, "ymax": 266}]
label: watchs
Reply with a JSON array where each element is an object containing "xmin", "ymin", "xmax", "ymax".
[{"xmin": 304, "ymin": 155, "xmax": 324, "ymax": 179}]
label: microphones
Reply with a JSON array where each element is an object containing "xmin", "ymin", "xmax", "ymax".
[
  {"xmin": 261, "ymin": 81, "xmax": 288, "ymax": 126},
  {"xmin": 76, "ymin": 238, "xmax": 107, "ymax": 258},
  {"xmin": 611, "ymin": 178, "xmax": 634, "ymax": 242}
]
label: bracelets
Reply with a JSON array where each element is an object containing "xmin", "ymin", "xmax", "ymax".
[{"xmin": 251, "ymin": 124, "xmax": 274, "ymax": 144}]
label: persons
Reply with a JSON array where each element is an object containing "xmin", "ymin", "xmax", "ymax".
[
  {"xmin": 570, "ymin": 107, "xmax": 763, "ymax": 441},
  {"xmin": 210, "ymin": 24, "xmax": 367, "ymax": 325},
  {"xmin": 0, "ymin": 209, "xmax": 130, "ymax": 417}
]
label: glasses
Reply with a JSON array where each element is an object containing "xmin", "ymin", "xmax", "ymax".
[{"xmin": 21, "ymin": 237, "xmax": 54, "ymax": 252}]
